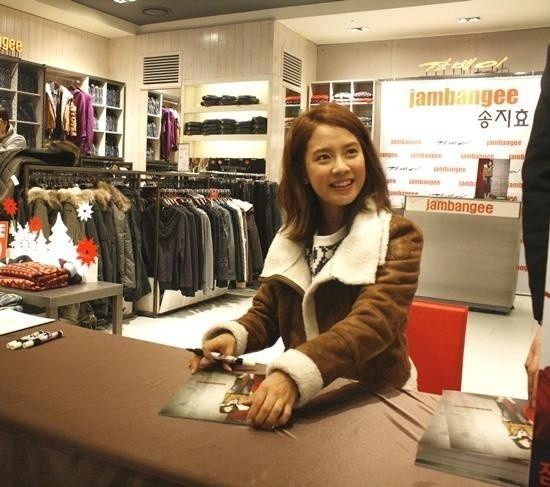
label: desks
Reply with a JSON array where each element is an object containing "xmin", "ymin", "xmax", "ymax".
[
  {"xmin": 0, "ymin": 311, "xmax": 535, "ymax": 487},
  {"xmin": 0, "ymin": 280, "xmax": 123, "ymax": 336}
]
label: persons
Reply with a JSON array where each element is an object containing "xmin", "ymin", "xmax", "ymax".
[
  {"xmin": 494, "ymin": 397, "xmax": 534, "ymax": 449},
  {"xmin": 521, "ymin": 44, "xmax": 550, "ymax": 410},
  {"xmin": 220, "ymin": 373, "xmax": 255, "ymax": 413},
  {"xmin": 482, "ymin": 160, "xmax": 494, "ymax": 199},
  {"xmin": 185, "ymin": 100, "xmax": 421, "ymax": 429},
  {"xmin": 0, "ymin": 104, "xmax": 27, "ymax": 151}
]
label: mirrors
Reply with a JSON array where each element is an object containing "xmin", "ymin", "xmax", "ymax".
[
  {"xmin": 138, "ymin": 82, "xmax": 184, "ymax": 172},
  {"xmin": 285, "ymin": 86, "xmax": 301, "ymax": 134}
]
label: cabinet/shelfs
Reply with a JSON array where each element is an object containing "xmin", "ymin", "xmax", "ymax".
[
  {"xmin": 181, "ymin": 76, "xmax": 282, "ymax": 173},
  {"xmin": 308, "ymin": 79, "xmax": 379, "ymax": 156},
  {"xmin": 146, "ymin": 89, "xmax": 181, "ymax": 171},
  {"xmin": 0, "ymin": 55, "xmax": 126, "ymax": 157}
]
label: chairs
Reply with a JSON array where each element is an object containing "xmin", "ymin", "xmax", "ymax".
[{"xmin": 405, "ymin": 299, "xmax": 468, "ymax": 394}]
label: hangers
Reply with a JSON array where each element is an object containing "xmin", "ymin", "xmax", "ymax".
[
  {"xmin": 160, "ymin": 188, "xmax": 231, "ymax": 209},
  {"xmin": 30, "ymin": 172, "xmax": 72, "ymax": 190}
]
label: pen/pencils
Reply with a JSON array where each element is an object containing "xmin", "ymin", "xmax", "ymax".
[
  {"xmin": 6, "ymin": 330, "xmax": 63, "ymax": 350},
  {"xmin": 185, "ymin": 348, "xmax": 244, "ymax": 366}
]
